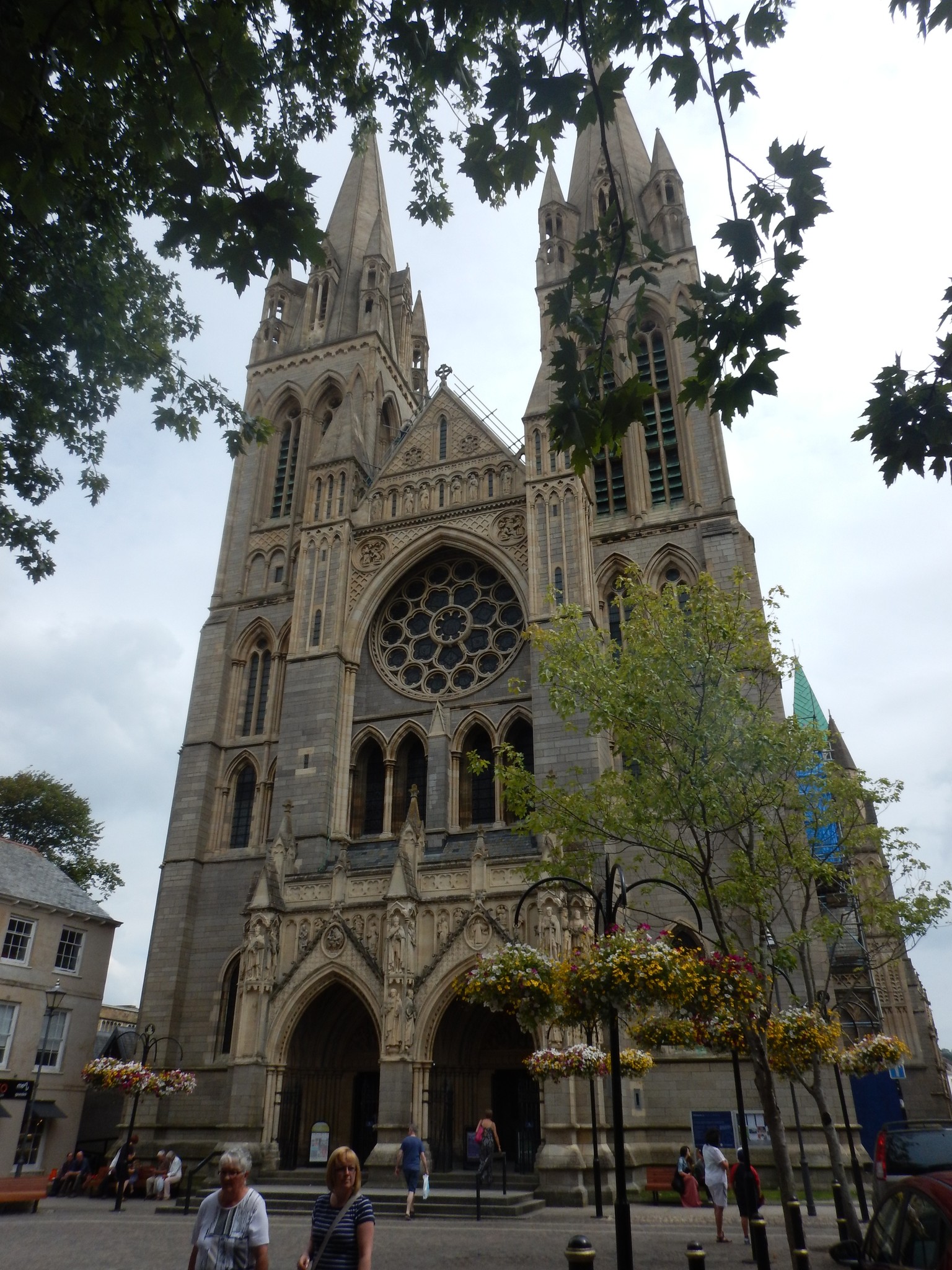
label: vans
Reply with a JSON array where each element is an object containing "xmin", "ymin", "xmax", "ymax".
[{"xmin": 871, "ymin": 1119, "xmax": 952, "ymax": 1215}]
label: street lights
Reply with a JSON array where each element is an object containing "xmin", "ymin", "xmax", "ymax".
[
  {"xmin": 16, "ymin": 978, "xmax": 68, "ymax": 1177},
  {"xmin": 514, "ymin": 853, "xmax": 704, "ymax": 1269},
  {"xmin": 99, "ymin": 1025, "xmax": 184, "ymax": 1211}
]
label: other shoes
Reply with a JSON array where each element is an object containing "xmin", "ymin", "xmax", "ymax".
[
  {"xmin": 47, "ymin": 1188, "xmax": 171, "ymax": 1201},
  {"xmin": 744, "ymin": 1235, "xmax": 750, "ymax": 1245},
  {"xmin": 487, "ymin": 1181, "xmax": 493, "ymax": 1187},
  {"xmin": 479, "ymin": 1179, "xmax": 483, "ymax": 1185}
]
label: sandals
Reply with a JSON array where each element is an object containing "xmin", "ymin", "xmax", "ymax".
[
  {"xmin": 716, "ymin": 1236, "xmax": 732, "ymax": 1243},
  {"xmin": 722, "ymin": 1231, "xmax": 724, "ymax": 1238}
]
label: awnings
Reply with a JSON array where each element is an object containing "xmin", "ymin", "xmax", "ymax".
[{"xmin": 26, "ymin": 1101, "xmax": 68, "ymax": 1120}]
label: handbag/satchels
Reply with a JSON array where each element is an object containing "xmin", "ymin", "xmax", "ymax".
[
  {"xmin": 671, "ymin": 1167, "xmax": 685, "ymax": 1195},
  {"xmin": 475, "ymin": 1119, "xmax": 484, "ymax": 1142},
  {"xmin": 423, "ymin": 1174, "xmax": 430, "ymax": 1199}
]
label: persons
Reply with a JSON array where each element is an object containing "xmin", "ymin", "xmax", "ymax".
[
  {"xmin": 687, "ymin": 1147, "xmax": 714, "ymax": 1205},
  {"xmin": 702, "ymin": 1129, "xmax": 732, "ymax": 1243},
  {"xmin": 395, "ymin": 1124, "xmax": 429, "ymax": 1220},
  {"xmin": 726, "ymin": 1147, "xmax": 762, "ymax": 1244},
  {"xmin": 155, "ymin": 1150, "xmax": 182, "ymax": 1202},
  {"xmin": 675, "ymin": 1145, "xmax": 703, "ymax": 1208},
  {"xmin": 296, "ymin": 1146, "xmax": 376, "ymax": 1270},
  {"xmin": 46, "ymin": 1151, "xmax": 77, "ymax": 1198},
  {"xmin": 95, "ymin": 1130, "xmax": 141, "ymax": 1202},
  {"xmin": 186, "ymin": 1145, "xmax": 270, "ymax": 1270},
  {"xmin": 475, "ymin": 1107, "xmax": 502, "ymax": 1187},
  {"xmin": 55, "ymin": 1151, "xmax": 92, "ymax": 1199},
  {"xmin": 145, "ymin": 1150, "xmax": 170, "ymax": 1201}
]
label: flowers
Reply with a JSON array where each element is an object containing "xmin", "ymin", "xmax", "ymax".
[
  {"xmin": 451, "ymin": 923, "xmax": 911, "ymax": 1084},
  {"xmin": 81, "ymin": 1056, "xmax": 197, "ymax": 1101}
]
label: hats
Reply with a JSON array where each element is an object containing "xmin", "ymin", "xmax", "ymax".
[{"xmin": 737, "ymin": 1147, "xmax": 744, "ymax": 1158}]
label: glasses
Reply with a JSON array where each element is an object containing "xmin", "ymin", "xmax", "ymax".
[
  {"xmin": 217, "ymin": 1168, "xmax": 246, "ymax": 1176},
  {"xmin": 334, "ymin": 1166, "xmax": 356, "ymax": 1174}
]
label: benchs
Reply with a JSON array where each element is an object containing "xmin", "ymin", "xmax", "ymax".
[
  {"xmin": 89, "ymin": 1167, "xmax": 188, "ymax": 1200},
  {"xmin": 644, "ymin": 1168, "xmax": 732, "ymax": 1204},
  {"xmin": 0, "ymin": 1176, "xmax": 46, "ymax": 1214}
]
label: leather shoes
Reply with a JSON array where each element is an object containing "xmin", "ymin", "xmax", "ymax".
[
  {"xmin": 405, "ymin": 1214, "xmax": 411, "ymax": 1220},
  {"xmin": 409, "ymin": 1210, "xmax": 414, "ymax": 1217}
]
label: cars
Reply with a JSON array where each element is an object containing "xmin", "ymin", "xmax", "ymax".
[{"xmin": 829, "ymin": 1171, "xmax": 952, "ymax": 1270}]
